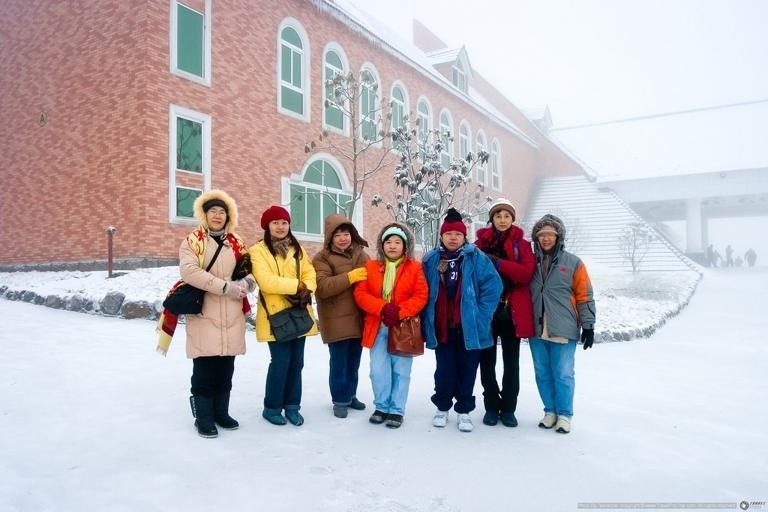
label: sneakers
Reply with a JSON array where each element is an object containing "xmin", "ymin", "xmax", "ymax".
[
  {"xmin": 431, "ymin": 409, "xmax": 450, "ymax": 428},
  {"xmin": 456, "ymin": 413, "xmax": 475, "ymax": 433},
  {"xmin": 554, "ymin": 414, "xmax": 572, "ymax": 434},
  {"xmin": 537, "ymin": 412, "xmax": 558, "ymax": 429}
]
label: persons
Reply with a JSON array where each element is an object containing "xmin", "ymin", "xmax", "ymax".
[
  {"xmin": 179, "ymin": 189, "xmax": 258, "ymax": 437},
  {"xmin": 312, "ymin": 214, "xmax": 372, "ymax": 418},
  {"xmin": 246, "ymin": 205, "xmax": 318, "ymax": 426},
  {"xmin": 354, "ymin": 222, "xmax": 429, "ymax": 428},
  {"xmin": 528, "ymin": 214, "xmax": 596, "ymax": 433},
  {"xmin": 472, "ymin": 197, "xmax": 536, "ymax": 428},
  {"xmin": 705, "ymin": 244, "xmax": 757, "ymax": 268},
  {"xmin": 420, "ymin": 207, "xmax": 504, "ymax": 432}
]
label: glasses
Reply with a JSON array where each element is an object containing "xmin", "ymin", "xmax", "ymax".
[{"xmin": 206, "ymin": 209, "xmax": 228, "ymax": 218}]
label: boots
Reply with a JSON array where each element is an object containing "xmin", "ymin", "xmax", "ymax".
[
  {"xmin": 369, "ymin": 401, "xmax": 389, "ymax": 426},
  {"xmin": 189, "ymin": 389, "xmax": 219, "ymax": 440},
  {"xmin": 213, "ymin": 385, "xmax": 239, "ymax": 432},
  {"xmin": 385, "ymin": 412, "xmax": 404, "ymax": 430},
  {"xmin": 284, "ymin": 386, "xmax": 306, "ymax": 427},
  {"xmin": 331, "ymin": 387, "xmax": 349, "ymax": 419},
  {"xmin": 482, "ymin": 399, "xmax": 500, "ymax": 427},
  {"xmin": 260, "ymin": 387, "xmax": 287, "ymax": 427},
  {"xmin": 500, "ymin": 398, "xmax": 519, "ymax": 428},
  {"xmin": 347, "ymin": 388, "xmax": 367, "ymax": 412}
]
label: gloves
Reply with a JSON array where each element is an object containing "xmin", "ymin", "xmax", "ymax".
[
  {"xmin": 285, "ymin": 279, "xmax": 314, "ymax": 310},
  {"xmin": 221, "ymin": 276, "xmax": 252, "ymax": 300},
  {"xmin": 482, "ymin": 254, "xmax": 502, "ymax": 271},
  {"xmin": 579, "ymin": 326, "xmax": 596, "ymax": 350},
  {"xmin": 346, "ymin": 266, "xmax": 369, "ymax": 285},
  {"xmin": 381, "ymin": 303, "xmax": 400, "ymax": 326}
]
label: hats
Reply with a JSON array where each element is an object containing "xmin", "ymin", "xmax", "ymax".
[
  {"xmin": 438, "ymin": 206, "xmax": 470, "ymax": 236},
  {"xmin": 259, "ymin": 204, "xmax": 292, "ymax": 230},
  {"xmin": 487, "ymin": 196, "xmax": 517, "ymax": 223},
  {"xmin": 380, "ymin": 226, "xmax": 409, "ymax": 246},
  {"xmin": 534, "ymin": 225, "xmax": 559, "ymax": 239}
]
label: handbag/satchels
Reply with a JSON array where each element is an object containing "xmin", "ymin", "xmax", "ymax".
[
  {"xmin": 264, "ymin": 304, "xmax": 314, "ymax": 343},
  {"xmin": 385, "ymin": 314, "xmax": 426, "ymax": 357},
  {"xmin": 162, "ymin": 282, "xmax": 208, "ymax": 316}
]
